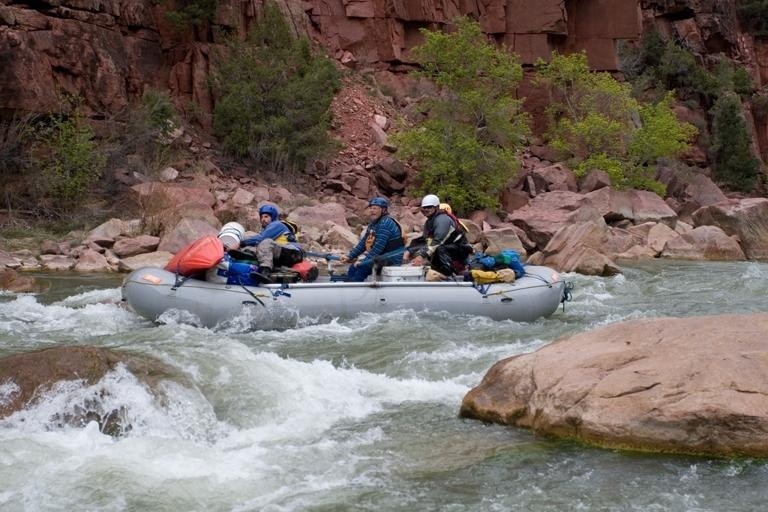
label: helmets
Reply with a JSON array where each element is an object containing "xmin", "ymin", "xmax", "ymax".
[
  {"xmin": 439, "ymin": 203, "xmax": 453, "ymax": 213},
  {"xmin": 369, "ymin": 197, "xmax": 388, "ymax": 209},
  {"xmin": 258, "ymin": 205, "xmax": 278, "ymax": 222},
  {"xmin": 420, "ymin": 194, "xmax": 438, "ymax": 208}
]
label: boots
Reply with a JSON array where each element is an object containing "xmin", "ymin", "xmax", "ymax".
[{"xmin": 249, "ymin": 265, "xmax": 273, "ymax": 285}]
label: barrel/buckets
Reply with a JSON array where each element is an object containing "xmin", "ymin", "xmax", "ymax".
[
  {"xmin": 217, "ymin": 222, "xmax": 245, "ymax": 251},
  {"xmin": 206, "ymin": 253, "xmax": 259, "ymax": 285}
]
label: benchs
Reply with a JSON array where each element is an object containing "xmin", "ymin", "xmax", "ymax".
[{"xmin": 346, "ymin": 264, "xmax": 423, "ymax": 283}]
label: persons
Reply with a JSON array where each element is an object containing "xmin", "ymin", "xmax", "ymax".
[
  {"xmin": 402, "ymin": 195, "xmax": 470, "ymax": 281},
  {"xmin": 238, "ymin": 205, "xmax": 303, "ymax": 278},
  {"xmin": 339, "ymin": 197, "xmax": 404, "ymax": 282}
]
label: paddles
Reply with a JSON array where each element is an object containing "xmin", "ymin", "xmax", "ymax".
[{"xmin": 357, "ymin": 235, "xmax": 428, "ymax": 267}]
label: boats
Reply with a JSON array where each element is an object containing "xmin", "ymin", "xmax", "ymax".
[{"xmin": 114, "ymin": 251, "xmax": 576, "ymax": 325}]
